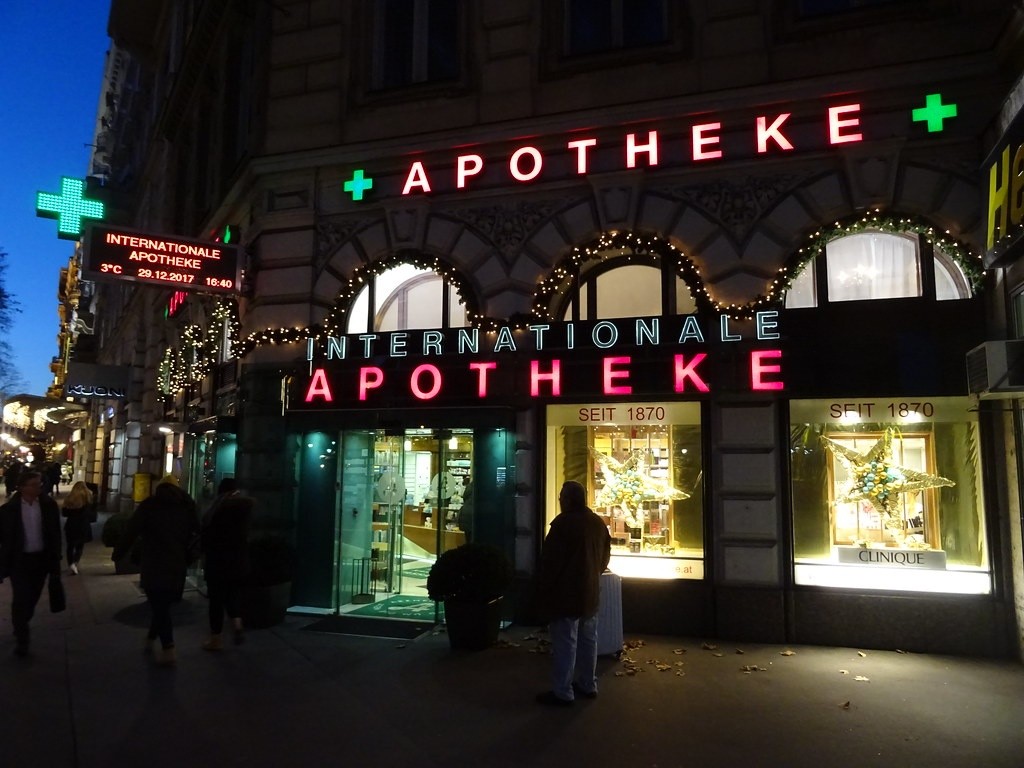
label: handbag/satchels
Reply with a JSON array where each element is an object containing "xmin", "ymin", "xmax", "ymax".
[{"xmin": 49, "ymin": 569, "xmax": 70, "ymax": 616}]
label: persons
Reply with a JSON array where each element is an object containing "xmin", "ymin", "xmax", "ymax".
[
  {"xmin": 124, "ymin": 475, "xmax": 257, "ymax": 670},
  {"xmin": 0, "ymin": 472, "xmax": 63, "ymax": 663},
  {"xmin": 530, "ymin": 481, "xmax": 611, "ymax": 709},
  {"xmin": 62, "ymin": 481, "xmax": 98, "ymax": 576}
]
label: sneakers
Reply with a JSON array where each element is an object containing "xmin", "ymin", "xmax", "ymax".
[{"xmin": 66, "ymin": 565, "xmax": 81, "ymax": 576}]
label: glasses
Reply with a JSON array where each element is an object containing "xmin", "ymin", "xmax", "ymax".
[{"xmin": 22, "ymin": 482, "xmax": 45, "ymax": 491}]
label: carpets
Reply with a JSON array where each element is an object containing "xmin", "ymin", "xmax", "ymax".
[{"xmin": 299, "ymin": 614, "xmax": 436, "ymax": 641}]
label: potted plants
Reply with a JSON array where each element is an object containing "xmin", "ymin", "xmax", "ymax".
[
  {"xmin": 237, "ymin": 537, "xmax": 302, "ymax": 627},
  {"xmin": 428, "ymin": 541, "xmax": 517, "ymax": 652},
  {"xmin": 102, "ymin": 511, "xmax": 141, "ymax": 574}
]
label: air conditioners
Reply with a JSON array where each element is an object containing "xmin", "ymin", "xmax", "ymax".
[{"xmin": 965, "ymin": 339, "xmax": 1024, "ymax": 398}]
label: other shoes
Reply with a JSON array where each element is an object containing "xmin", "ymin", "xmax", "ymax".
[
  {"xmin": 201, "ymin": 635, "xmax": 226, "ymax": 655},
  {"xmin": 16, "ymin": 634, "xmax": 31, "ymax": 654},
  {"xmin": 161, "ymin": 650, "xmax": 179, "ymax": 663},
  {"xmin": 135, "ymin": 635, "xmax": 155, "ymax": 650},
  {"xmin": 538, "ymin": 690, "xmax": 574, "ymax": 707},
  {"xmin": 566, "ymin": 681, "xmax": 599, "ymax": 699},
  {"xmin": 230, "ymin": 630, "xmax": 248, "ymax": 649}
]
